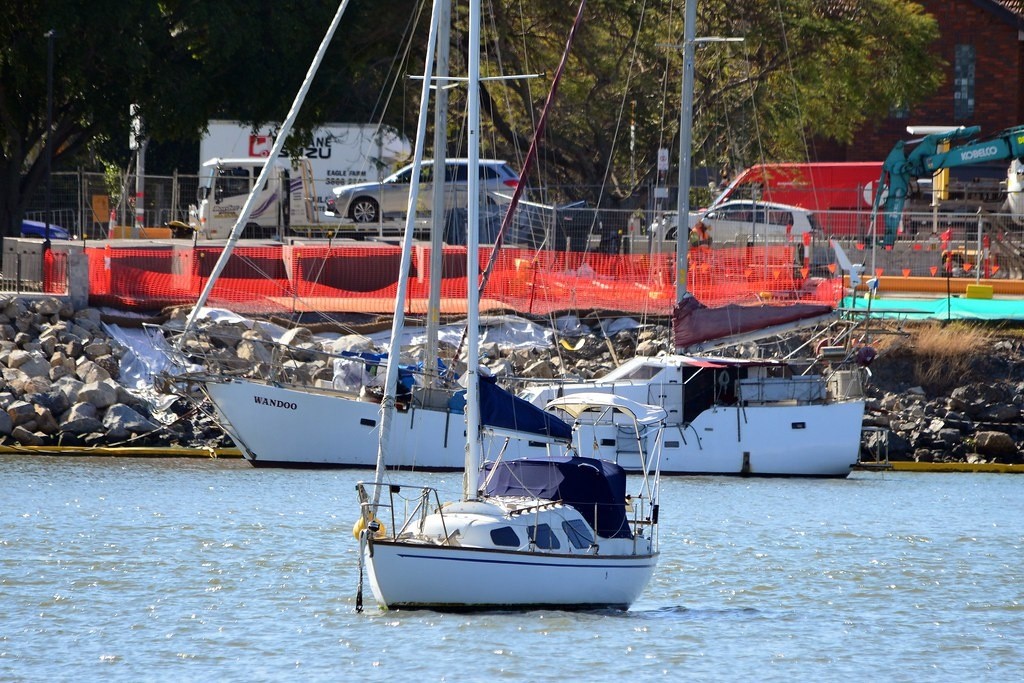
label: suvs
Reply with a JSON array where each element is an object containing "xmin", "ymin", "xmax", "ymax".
[
  {"xmin": 323, "ymin": 157, "xmax": 527, "ymax": 223},
  {"xmin": 654, "ymin": 199, "xmax": 815, "ymax": 239}
]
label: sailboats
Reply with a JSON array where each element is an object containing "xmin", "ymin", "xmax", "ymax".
[
  {"xmin": 351, "ymin": 0, "xmax": 708, "ymax": 615},
  {"xmin": 158, "ymin": 1, "xmax": 873, "ymax": 482}
]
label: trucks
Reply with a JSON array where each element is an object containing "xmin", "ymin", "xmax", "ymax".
[{"xmin": 195, "ymin": 156, "xmax": 448, "ymax": 240}]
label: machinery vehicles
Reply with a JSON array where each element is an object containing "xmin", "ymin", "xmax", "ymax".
[{"xmin": 860, "ymin": 124, "xmax": 1024, "ymax": 243}]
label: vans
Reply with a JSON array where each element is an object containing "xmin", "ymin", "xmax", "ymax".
[{"xmin": 709, "ymin": 160, "xmax": 908, "ymax": 238}]
